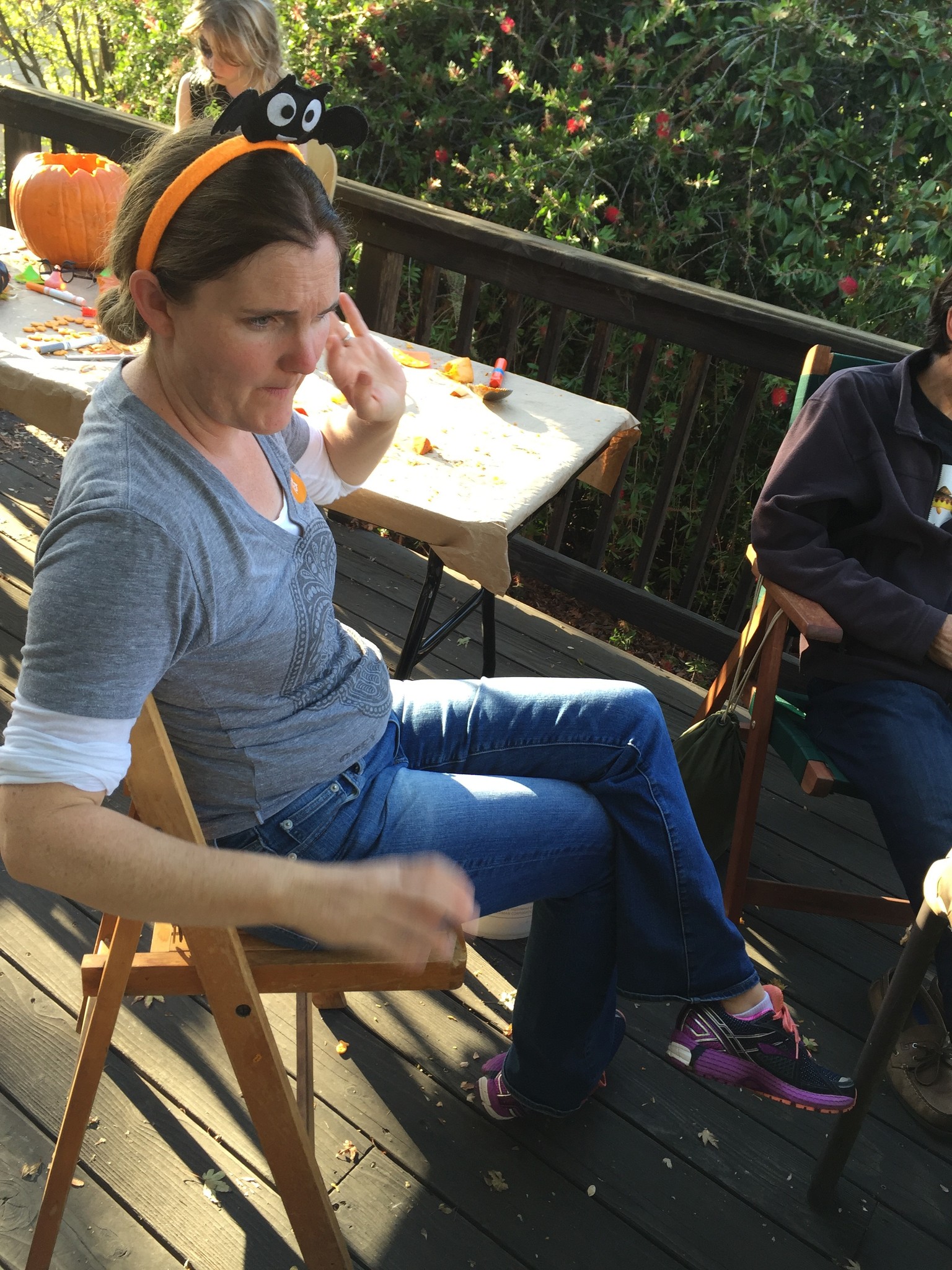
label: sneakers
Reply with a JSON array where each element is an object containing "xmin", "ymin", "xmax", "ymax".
[
  {"xmin": 665, "ymin": 985, "xmax": 856, "ymax": 1115},
  {"xmin": 475, "ymin": 1007, "xmax": 630, "ymax": 1123},
  {"xmin": 867, "ymin": 966, "xmax": 952, "ymax": 1125}
]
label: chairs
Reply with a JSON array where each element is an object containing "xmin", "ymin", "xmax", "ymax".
[
  {"xmin": 687, "ymin": 345, "xmax": 915, "ymax": 934},
  {"xmin": 27, "ymin": 689, "xmax": 485, "ymax": 1268}
]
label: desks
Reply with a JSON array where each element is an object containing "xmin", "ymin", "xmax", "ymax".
[{"xmin": 0, "ymin": 222, "xmax": 640, "ymax": 680}]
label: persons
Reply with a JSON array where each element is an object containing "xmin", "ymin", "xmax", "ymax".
[
  {"xmin": 174, "ymin": 0, "xmax": 307, "ymax": 162},
  {"xmin": 0, "ymin": 74, "xmax": 857, "ymax": 1120},
  {"xmin": 751, "ymin": 266, "xmax": 952, "ymax": 1137}
]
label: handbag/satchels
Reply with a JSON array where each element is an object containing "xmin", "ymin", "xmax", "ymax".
[{"xmin": 670, "ymin": 710, "xmax": 747, "ymax": 894}]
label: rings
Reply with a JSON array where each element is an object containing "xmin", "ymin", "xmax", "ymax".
[{"xmin": 341, "ymin": 333, "xmax": 351, "ymax": 343}]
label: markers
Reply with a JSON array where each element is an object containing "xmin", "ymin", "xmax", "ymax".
[
  {"xmin": 490, "ymin": 358, "xmax": 507, "ymax": 387},
  {"xmin": 26, "ymin": 281, "xmax": 86, "ymax": 306},
  {"xmin": 38, "ymin": 334, "xmax": 108, "ymax": 355}
]
